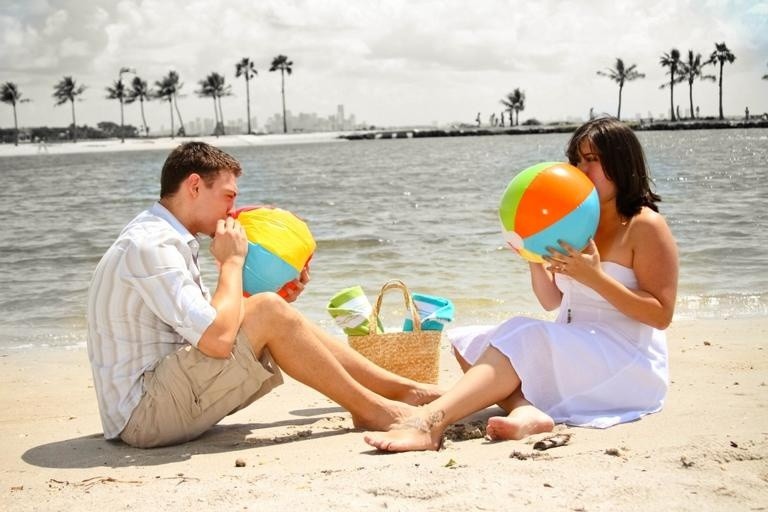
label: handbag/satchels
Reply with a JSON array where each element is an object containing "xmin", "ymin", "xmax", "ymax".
[{"xmin": 346, "ymin": 279, "xmax": 442, "ymax": 386}]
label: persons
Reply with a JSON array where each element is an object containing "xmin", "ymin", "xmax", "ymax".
[
  {"xmin": 83, "ymin": 137, "xmax": 443, "ymax": 451},
  {"xmin": 361, "ymin": 113, "xmax": 679, "ymax": 455}
]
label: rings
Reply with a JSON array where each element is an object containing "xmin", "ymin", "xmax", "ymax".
[{"xmin": 559, "ymin": 263, "xmax": 565, "ymax": 272}]
